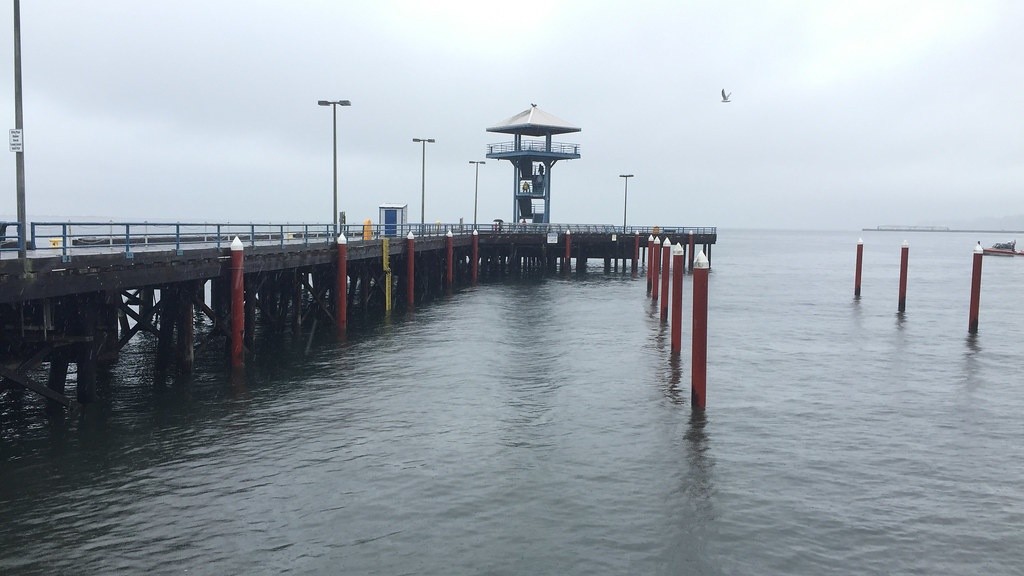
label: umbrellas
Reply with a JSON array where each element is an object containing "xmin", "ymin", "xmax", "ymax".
[{"xmin": 493, "ymin": 219, "xmax": 503, "ymax": 222}]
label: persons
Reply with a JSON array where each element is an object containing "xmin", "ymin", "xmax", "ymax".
[
  {"xmin": 522, "ymin": 218, "xmax": 526, "ymax": 223},
  {"xmin": 523, "ymin": 182, "xmax": 529, "ymax": 192},
  {"xmin": 538, "ymin": 164, "xmax": 544, "ymax": 176},
  {"xmin": 498, "ymin": 222, "xmax": 502, "ymax": 233}
]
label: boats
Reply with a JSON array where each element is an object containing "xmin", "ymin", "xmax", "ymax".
[{"xmin": 982, "ymin": 239, "xmax": 1024, "ymax": 258}]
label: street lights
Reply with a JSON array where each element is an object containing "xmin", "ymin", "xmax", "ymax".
[
  {"xmin": 412, "ymin": 138, "xmax": 435, "ymax": 235},
  {"xmin": 620, "ymin": 174, "xmax": 634, "ymax": 236},
  {"xmin": 469, "ymin": 159, "xmax": 486, "ymax": 231},
  {"xmin": 317, "ymin": 99, "xmax": 352, "ymax": 235}
]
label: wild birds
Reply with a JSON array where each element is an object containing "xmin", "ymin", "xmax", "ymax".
[{"xmin": 721, "ymin": 88, "xmax": 732, "ymax": 102}]
